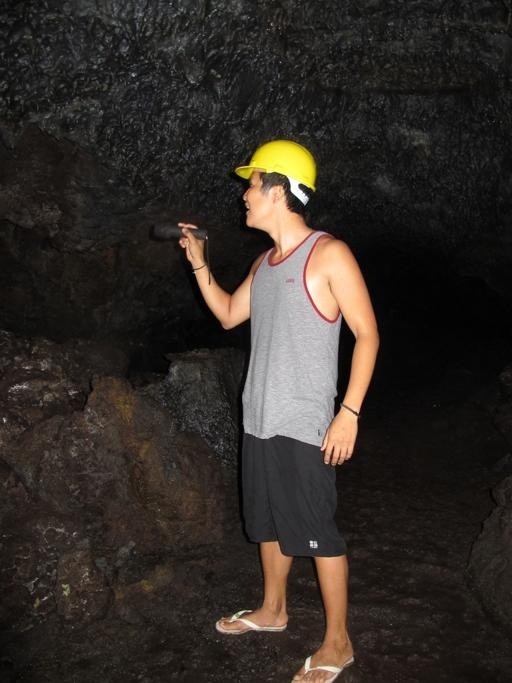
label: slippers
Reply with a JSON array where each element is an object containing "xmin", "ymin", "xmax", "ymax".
[
  {"xmin": 291, "ymin": 649, "xmax": 356, "ymax": 682},
  {"xmin": 214, "ymin": 608, "xmax": 289, "ymax": 636}
]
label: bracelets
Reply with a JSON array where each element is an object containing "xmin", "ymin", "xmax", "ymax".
[
  {"xmin": 340, "ymin": 403, "xmax": 361, "ymax": 419},
  {"xmin": 192, "ymin": 263, "xmax": 207, "ymax": 272}
]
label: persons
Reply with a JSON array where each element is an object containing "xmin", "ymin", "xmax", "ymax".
[{"xmin": 179, "ymin": 140, "xmax": 379, "ymax": 683}]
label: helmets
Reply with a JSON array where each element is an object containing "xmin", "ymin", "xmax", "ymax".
[{"xmin": 234, "ymin": 139, "xmax": 318, "ymax": 207}]
